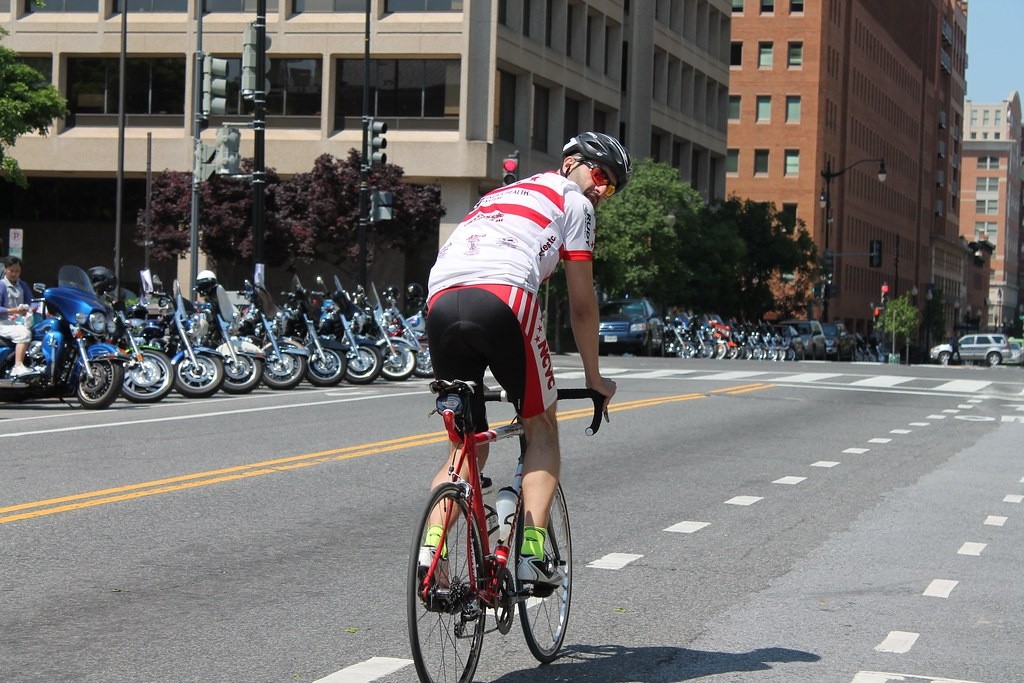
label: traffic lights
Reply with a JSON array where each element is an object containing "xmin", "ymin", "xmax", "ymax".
[
  {"xmin": 196, "ymin": 144, "xmax": 217, "ymax": 183},
  {"xmin": 503, "ymin": 158, "xmax": 519, "ymax": 186},
  {"xmin": 240, "ymin": 23, "xmax": 272, "ymax": 100},
  {"xmin": 872, "ymin": 309, "xmax": 879, "ymax": 324},
  {"xmin": 368, "ymin": 117, "xmax": 387, "ymax": 167},
  {"xmin": 201, "ymin": 53, "xmax": 229, "ymax": 118},
  {"xmin": 370, "ymin": 191, "xmax": 394, "ymax": 224},
  {"xmin": 881, "ymin": 284, "xmax": 888, "ymax": 304}
]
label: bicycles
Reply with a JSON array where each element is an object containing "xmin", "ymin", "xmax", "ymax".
[{"xmin": 406, "ymin": 378, "xmax": 611, "ymax": 683}]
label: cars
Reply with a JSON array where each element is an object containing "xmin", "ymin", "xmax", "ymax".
[
  {"xmin": 776, "ymin": 326, "xmax": 807, "ymax": 359},
  {"xmin": 1002, "ymin": 340, "xmax": 1024, "ymax": 366}
]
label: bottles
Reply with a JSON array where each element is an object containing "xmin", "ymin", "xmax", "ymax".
[
  {"xmin": 479, "ymin": 473, "xmax": 500, "ymax": 545},
  {"xmin": 494, "ymin": 485, "xmax": 519, "ymax": 549}
]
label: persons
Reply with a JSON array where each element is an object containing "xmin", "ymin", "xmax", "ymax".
[
  {"xmin": 0, "ymin": 257, "xmax": 37, "ymax": 378},
  {"xmin": 419, "ymin": 130, "xmax": 632, "ymax": 615},
  {"xmin": 948, "ymin": 331, "xmax": 964, "ymax": 365}
]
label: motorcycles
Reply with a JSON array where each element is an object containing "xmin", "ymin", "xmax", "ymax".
[
  {"xmin": 664, "ymin": 309, "xmax": 797, "ymax": 362},
  {"xmin": 851, "ymin": 334, "xmax": 886, "ymax": 362},
  {"xmin": 0, "ymin": 264, "xmax": 434, "ymax": 409}
]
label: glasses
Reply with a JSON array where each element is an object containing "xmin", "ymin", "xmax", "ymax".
[{"xmin": 581, "ymin": 160, "xmax": 616, "ymax": 199}]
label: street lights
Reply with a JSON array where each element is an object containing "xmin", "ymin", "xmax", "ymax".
[{"xmin": 819, "ymin": 157, "xmax": 889, "ymax": 317}]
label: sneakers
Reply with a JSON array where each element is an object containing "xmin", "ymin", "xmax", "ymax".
[
  {"xmin": 10, "ymin": 363, "xmax": 33, "ymax": 375},
  {"xmin": 517, "ymin": 554, "xmax": 565, "ymax": 587},
  {"xmin": 416, "ymin": 545, "xmax": 450, "ymax": 612}
]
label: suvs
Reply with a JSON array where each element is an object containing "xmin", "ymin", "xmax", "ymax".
[
  {"xmin": 780, "ymin": 319, "xmax": 828, "ymax": 360},
  {"xmin": 929, "ymin": 334, "xmax": 1013, "ymax": 366},
  {"xmin": 596, "ymin": 297, "xmax": 666, "ymax": 357},
  {"xmin": 820, "ymin": 321, "xmax": 855, "ymax": 361}
]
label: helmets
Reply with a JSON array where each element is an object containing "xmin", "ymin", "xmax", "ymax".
[
  {"xmin": 387, "ymin": 286, "xmax": 400, "ymax": 301},
  {"xmin": 406, "ymin": 283, "xmax": 423, "ymax": 299},
  {"xmin": 562, "ymin": 131, "xmax": 633, "ymax": 197},
  {"xmin": 92, "ymin": 268, "xmax": 117, "ymax": 292},
  {"xmin": 198, "ymin": 270, "xmax": 218, "ymax": 293}
]
